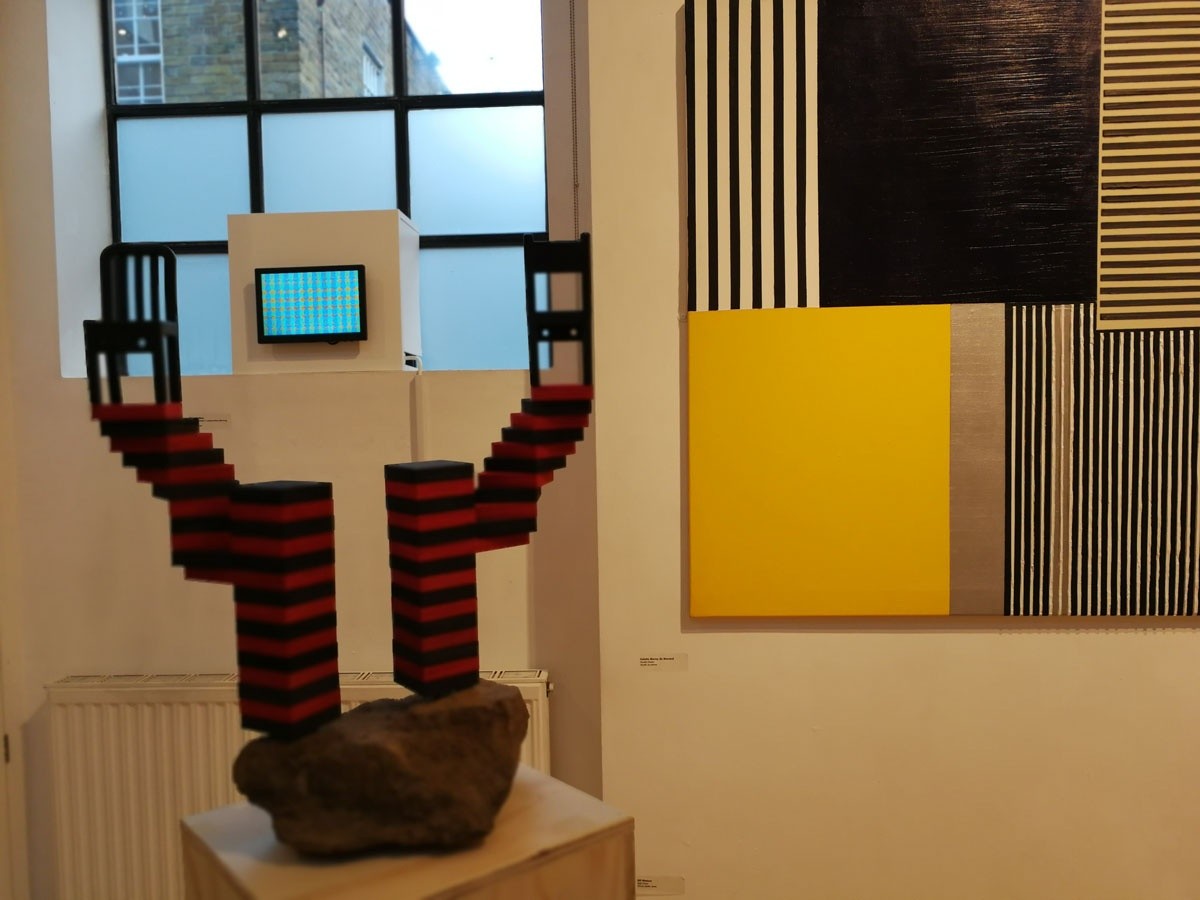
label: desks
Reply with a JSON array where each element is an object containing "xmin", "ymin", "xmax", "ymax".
[{"xmin": 179, "ymin": 761, "xmax": 636, "ymax": 900}]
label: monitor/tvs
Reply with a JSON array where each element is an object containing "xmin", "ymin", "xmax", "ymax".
[{"xmin": 255, "ymin": 265, "xmax": 368, "ymax": 344}]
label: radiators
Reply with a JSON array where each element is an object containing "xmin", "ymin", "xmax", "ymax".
[{"xmin": 46, "ymin": 670, "xmax": 555, "ymax": 900}]
